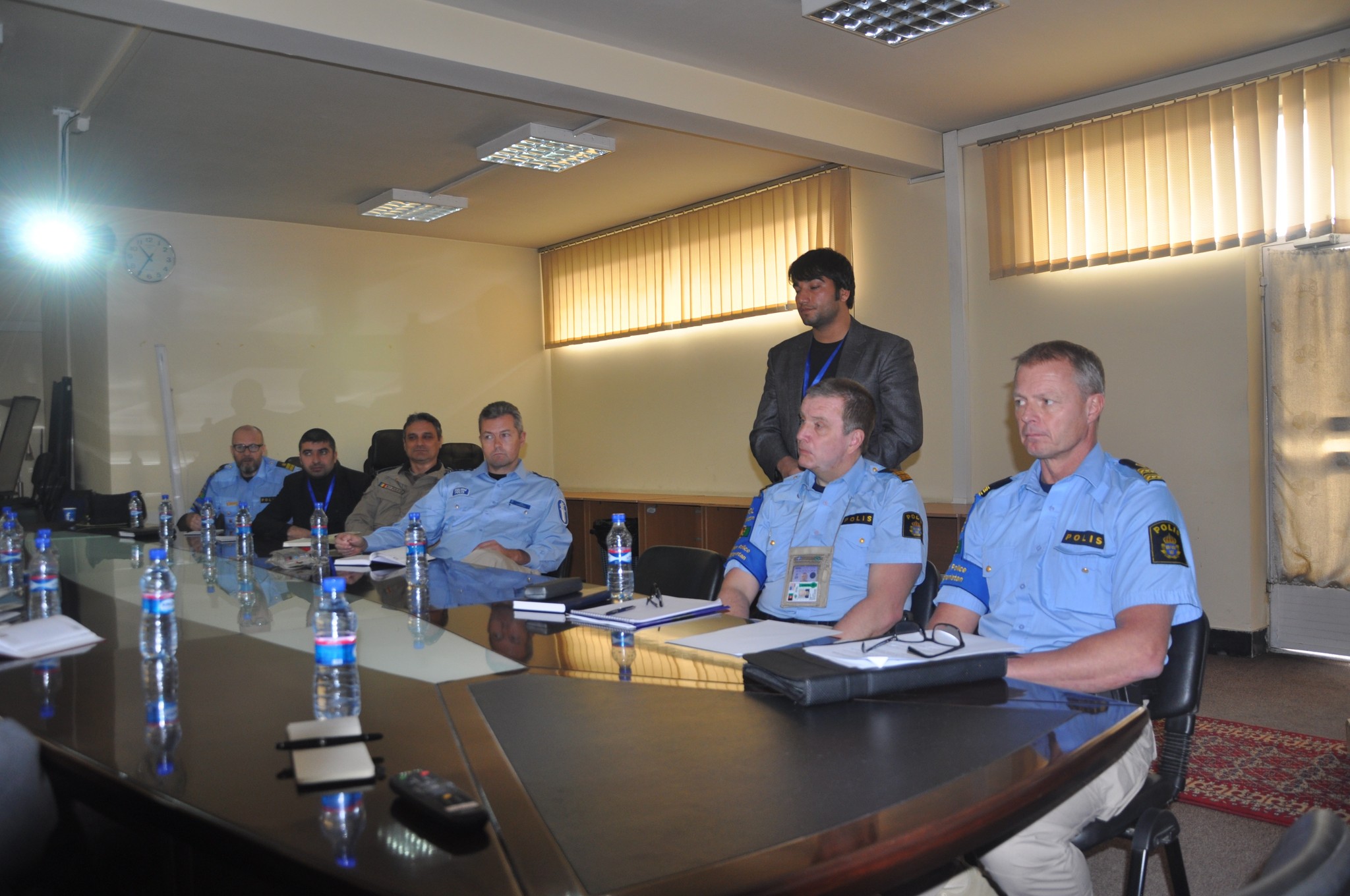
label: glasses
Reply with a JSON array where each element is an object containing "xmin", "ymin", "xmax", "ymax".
[
  {"xmin": 233, "ymin": 444, "xmax": 264, "ymax": 453},
  {"xmin": 861, "ymin": 620, "xmax": 965, "ymax": 658}
]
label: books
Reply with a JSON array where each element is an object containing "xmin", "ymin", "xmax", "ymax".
[
  {"xmin": 511, "ymin": 591, "xmax": 611, "ymax": 614},
  {"xmin": 566, "ymin": 597, "xmax": 731, "ymax": 633},
  {"xmin": 115, "ymin": 527, "xmax": 178, "ymax": 539},
  {"xmin": 283, "ymin": 531, "xmax": 363, "ymax": 548},
  {"xmin": 0, "ymin": 584, "xmax": 109, "ymax": 659},
  {"xmin": 0, "ymin": 643, "xmax": 100, "ymax": 673},
  {"xmin": 334, "ymin": 565, "xmax": 394, "ymax": 571},
  {"xmin": 513, "ymin": 610, "xmax": 569, "ymax": 623},
  {"xmin": 334, "ymin": 546, "xmax": 438, "ymax": 565}
]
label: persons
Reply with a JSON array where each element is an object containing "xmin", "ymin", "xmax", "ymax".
[
  {"xmin": 362, "ymin": 571, "xmax": 448, "ymax": 644},
  {"xmin": 283, "ymin": 569, "xmax": 374, "ymax": 626},
  {"xmin": 423, "ymin": 558, "xmax": 556, "ymax": 665},
  {"xmin": 334, "ymin": 402, "xmax": 573, "ymax": 577},
  {"xmin": 250, "ymin": 428, "xmax": 371, "ymax": 541},
  {"xmin": 175, "ymin": 425, "xmax": 302, "ymax": 536},
  {"xmin": 344, "ymin": 412, "xmax": 453, "ymax": 536},
  {"xmin": 715, "ymin": 376, "xmax": 929, "ymax": 646},
  {"xmin": 749, "ymin": 247, "xmax": 923, "ymax": 483},
  {"xmin": 912, "ymin": 340, "xmax": 1200, "ymax": 895},
  {"xmin": 183, "ymin": 536, "xmax": 294, "ymax": 633}
]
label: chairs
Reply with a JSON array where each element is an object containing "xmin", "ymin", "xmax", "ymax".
[
  {"xmin": 1231, "ymin": 810, "xmax": 1350, "ymax": 896},
  {"xmin": 1072, "ymin": 609, "xmax": 1214, "ymax": 895},
  {"xmin": 634, "ymin": 546, "xmax": 723, "ymax": 605}
]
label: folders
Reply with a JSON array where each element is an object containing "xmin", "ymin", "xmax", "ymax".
[{"xmin": 742, "ymin": 624, "xmax": 1006, "ymax": 706}]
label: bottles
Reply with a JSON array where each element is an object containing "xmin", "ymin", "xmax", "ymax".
[
  {"xmin": 157, "ymin": 494, "xmax": 176, "ymax": 546},
  {"xmin": 203, "ymin": 546, "xmax": 216, "ymax": 593},
  {"xmin": 308, "ymin": 501, "xmax": 330, "ymax": 561},
  {"xmin": 613, "ymin": 633, "xmax": 639, "ymax": 683},
  {"xmin": 319, "ymin": 795, "xmax": 363, "ymax": 873},
  {"xmin": 128, "ymin": 491, "xmax": 146, "ymax": 536},
  {"xmin": 405, "ymin": 590, "xmax": 431, "ymax": 650},
  {"xmin": 315, "ymin": 578, "xmax": 365, "ymax": 722},
  {"xmin": 142, "ymin": 543, "xmax": 179, "ymax": 664},
  {"xmin": 198, "ymin": 498, "xmax": 219, "ymax": 548},
  {"xmin": 606, "ymin": 513, "xmax": 640, "ymax": 606},
  {"xmin": 142, "ymin": 654, "xmax": 182, "ymax": 781},
  {"xmin": 236, "ymin": 505, "xmax": 254, "ymax": 560},
  {"xmin": 239, "ymin": 560, "xmax": 259, "ymax": 628},
  {"xmin": 405, "ymin": 514, "xmax": 428, "ymax": 586},
  {"xmin": 32, "ymin": 664, "xmax": 64, "ymax": 723},
  {"xmin": 131, "ymin": 542, "xmax": 142, "ymax": 571},
  {"xmin": 0, "ymin": 506, "xmax": 60, "ymax": 615}
]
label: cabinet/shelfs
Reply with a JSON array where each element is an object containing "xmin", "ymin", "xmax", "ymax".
[{"xmin": 549, "ymin": 493, "xmax": 988, "ymax": 607}]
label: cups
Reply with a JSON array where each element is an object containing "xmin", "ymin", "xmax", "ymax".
[{"xmin": 64, "ymin": 506, "xmax": 78, "ymax": 525}]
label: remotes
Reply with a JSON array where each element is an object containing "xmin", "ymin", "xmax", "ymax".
[{"xmin": 389, "ymin": 769, "xmax": 480, "ymax": 822}]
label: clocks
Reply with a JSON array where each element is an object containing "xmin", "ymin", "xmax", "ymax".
[{"xmin": 123, "ymin": 231, "xmax": 176, "ymax": 285}]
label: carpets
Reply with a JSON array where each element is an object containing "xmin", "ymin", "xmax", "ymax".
[{"xmin": 1144, "ymin": 707, "xmax": 1349, "ymax": 829}]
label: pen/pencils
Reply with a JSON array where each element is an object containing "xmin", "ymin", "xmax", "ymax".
[
  {"xmin": 605, "ymin": 606, "xmax": 636, "ymax": 616},
  {"xmin": 193, "ymin": 503, "xmax": 201, "ymax": 516},
  {"xmin": 275, "ymin": 733, "xmax": 384, "ymax": 749}
]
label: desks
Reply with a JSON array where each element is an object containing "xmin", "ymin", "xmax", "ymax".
[{"xmin": 0, "ymin": 530, "xmax": 1157, "ymax": 896}]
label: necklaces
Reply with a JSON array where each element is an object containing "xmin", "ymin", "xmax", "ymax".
[{"xmin": 305, "ymin": 475, "xmax": 335, "ymax": 514}]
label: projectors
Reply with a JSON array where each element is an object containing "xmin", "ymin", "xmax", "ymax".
[{"xmin": 12, "ymin": 204, "xmax": 98, "ymax": 272}]
